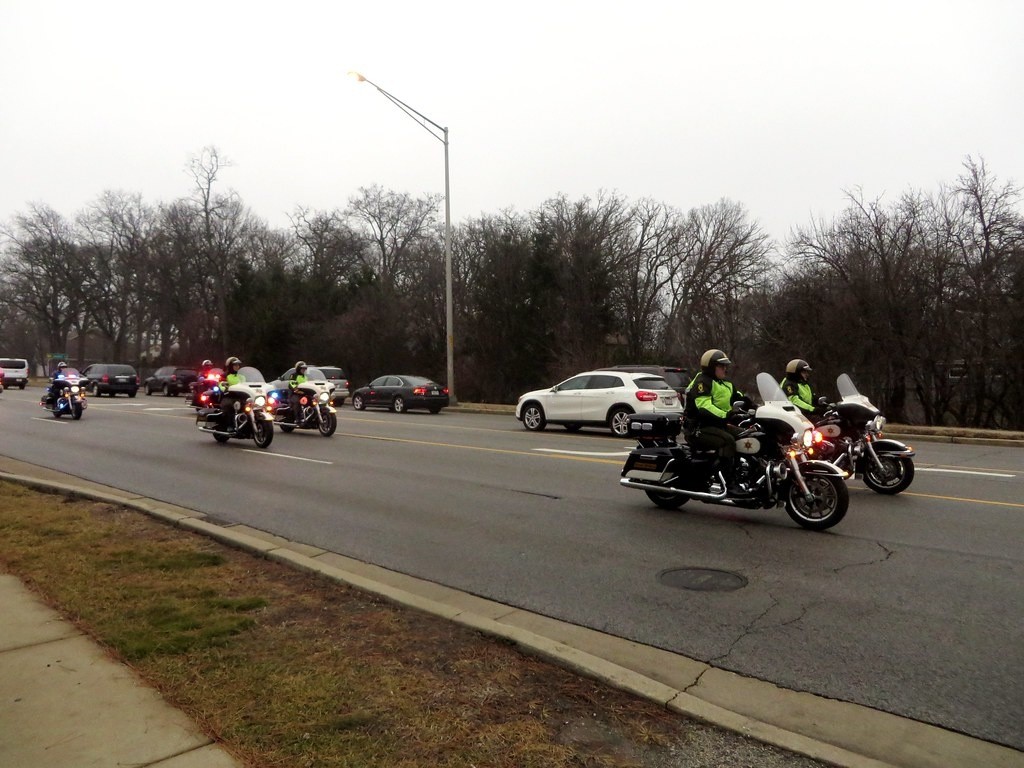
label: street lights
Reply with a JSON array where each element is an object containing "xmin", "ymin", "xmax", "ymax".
[{"xmin": 344, "ymin": 71, "xmax": 457, "ymax": 404}]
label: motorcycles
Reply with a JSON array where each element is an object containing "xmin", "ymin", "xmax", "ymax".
[
  {"xmin": 620, "ymin": 373, "xmax": 851, "ymax": 532},
  {"xmin": 814, "ymin": 373, "xmax": 918, "ymax": 496},
  {"xmin": 184, "ymin": 365, "xmax": 279, "ymax": 450},
  {"xmin": 273, "ymin": 368, "xmax": 338, "ymax": 437},
  {"xmin": 40, "ymin": 367, "xmax": 91, "ymax": 420}
]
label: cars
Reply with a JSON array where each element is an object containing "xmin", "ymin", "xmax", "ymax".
[
  {"xmin": 351, "ymin": 374, "xmax": 449, "ymax": 414},
  {"xmin": 144, "ymin": 366, "xmax": 200, "ymax": 396},
  {"xmin": 81, "ymin": 363, "xmax": 140, "ymax": 398}
]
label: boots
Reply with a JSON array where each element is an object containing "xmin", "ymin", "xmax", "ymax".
[{"xmin": 723, "ymin": 467, "xmax": 751, "ymax": 498}]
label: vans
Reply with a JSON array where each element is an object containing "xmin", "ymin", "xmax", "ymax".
[{"xmin": 0, "ymin": 358, "xmax": 29, "ymax": 388}]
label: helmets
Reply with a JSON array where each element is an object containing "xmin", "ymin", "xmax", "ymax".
[
  {"xmin": 700, "ymin": 349, "xmax": 732, "ymax": 367},
  {"xmin": 785, "ymin": 358, "xmax": 812, "ymax": 374},
  {"xmin": 57, "ymin": 362, "xmax": 68, "ymax": 368},
  {"xmin": 295, "ymin": 360, "xmax": 307, "ymax": 370},
  {"xmin": 202, "ymin": 360, "xmax": 213, "ymax": 366},
  {"xmin": 225, "ymin": 356, "xmax": 242, "ymax": 367}
]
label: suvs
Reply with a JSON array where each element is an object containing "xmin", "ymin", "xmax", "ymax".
[
  {"xmin": 268, "ymin": 364, "xmax": 349, "ymax": 407},
  {"xmin": 515, "ymin": 368, "xmax": 682, "ymax": 438}
]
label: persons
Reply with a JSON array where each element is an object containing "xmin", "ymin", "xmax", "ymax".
[
  {"xmin": 218, "ymin": 356, "xmax": 246, "ymax": 432},
  {"xmin": 779, "ymin": 359, "xmax": 826, "ymax": 419},
  {"xmin": 50, "ymin": 363, "xmax": 68, "ymax": 381},
  {"xmin": 197, "ymin": 360, "xmax": 212, "ymax": 387},
  {"xmin": 683, "ymin": 350, "xmax": 762, "ymax": 498},
  {"xmin": 288, "ymin": 361, "xmax": 309, "ymax": 426}
]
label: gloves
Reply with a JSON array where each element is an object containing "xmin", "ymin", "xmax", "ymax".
[
  {"xmin": 813, "ymin": 407, "xmax": 822, "ymax": 415},
  {"xmin": 726, "ymin": 410, "xmax": 744, "ymax": 424}
]
